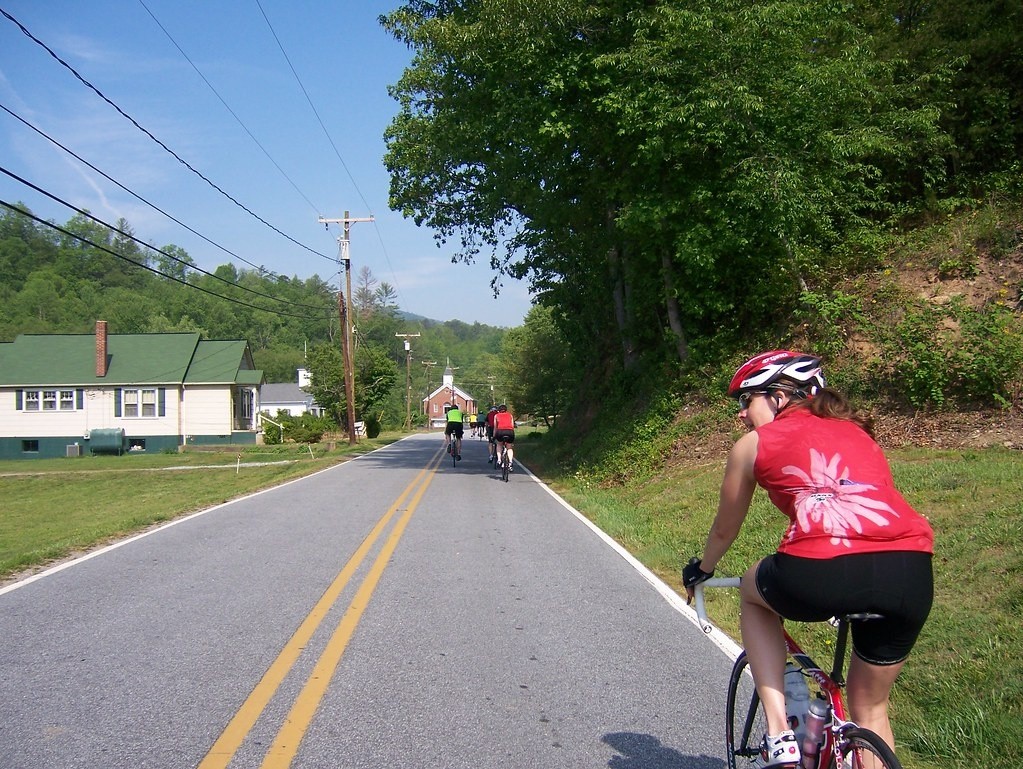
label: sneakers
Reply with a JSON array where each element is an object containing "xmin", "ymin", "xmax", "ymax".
[{"xmin": 751, "ymin": 729, "xmax": 801, "ymax": 769}]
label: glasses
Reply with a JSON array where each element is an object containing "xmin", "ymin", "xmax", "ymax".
[{"xmin": 738, "ymin": 391, "xmax": 768, "ymax": 411}]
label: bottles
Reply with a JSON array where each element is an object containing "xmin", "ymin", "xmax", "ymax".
[
  {"xmin": 802, "ymin": 692, "xmax": 827, "ymax": 769},
  {"xmin": 783, "ymin": 661, "xmax": 810, "ymax": 752}
]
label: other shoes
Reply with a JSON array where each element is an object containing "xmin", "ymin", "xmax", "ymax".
[
  {"xmin": 496, "ymin": 460, "xmax": 502, "ymax": 469},
  {"xmin": 488, "ymin": 455, "xmax": 493, "ymax": 461},
  {"xmin": 509, "ymin": 462, "xmax": 514, "ymax": 472},
  {"xmin": 447, "ymin": 444, "xmax": 451, "ymax": 453},
  {"xmin": 457, "ymin": 455, "xmax": 461, "ymax": 461}
]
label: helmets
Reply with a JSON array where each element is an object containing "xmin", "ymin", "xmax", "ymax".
[
  {"xmin": 728, "ymin": 351, "xmax": 824, "ymax": 399},
  {"xmin": 497, "ymin": 404, "xmax": 507, "ymax": 411},
  {"xmin": 489, "ymin": 405, "xmax": 497, "ymax": 410},
  {"xmin": 479, "ymin": 411, "xmax": 483, "ymax": 414}
]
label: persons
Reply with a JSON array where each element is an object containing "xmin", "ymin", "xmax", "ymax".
[
  {"xmin": 485, "ymin": 406, "xmax": 498, "ymax": 463},
  {"xmin": 681, "ymin": 350, "xmax": 934, "ymax": 769},
  {"xmin": 492, "ymin": 405, "xmax": 515, "ymax": 472},
  {"xmin": 476, "ymin": 411, "xmax": 485, "ymax": 437},
  {"xmin": 445, "ymin": 405, "xmax": 464, "ymax": 460},
  {"xmin": 469, "ymin": 413, "xmax": 477, "ymax": 434}
]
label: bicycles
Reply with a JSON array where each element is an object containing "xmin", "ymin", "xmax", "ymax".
[
  {"xmin": 490, "ymin": 435, "xmax": 509, "ymax": 482},
  {"xmin": 472, "ymin": 424, "xmax": 484, "ymax": 441},
  {"xmin": 450, "ymin": 432, "xmax": 457, "ymax": 468},
  {"xmin": 687, "ymin": 555, "xmax": 903, "ymax": 769}
]
